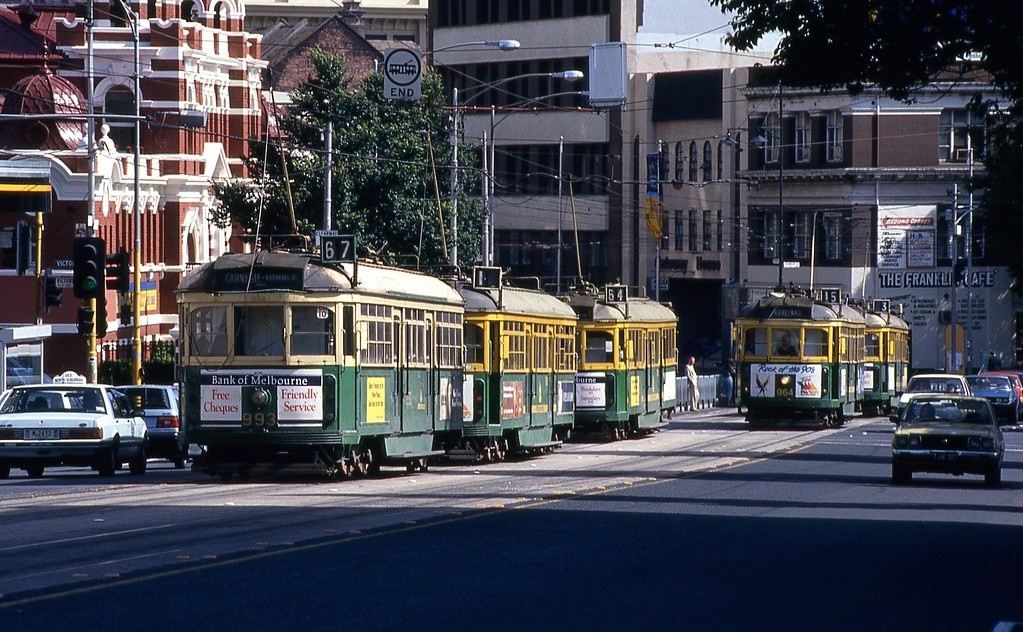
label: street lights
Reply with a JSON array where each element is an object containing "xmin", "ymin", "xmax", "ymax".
[
  {"xmin": 133, "ymin": 109, "xmax": 207, "ymax": 384},
  {"xmin": 723, "ymin": 126, "xmax": 784, "ymax": 283},
  {"xmin": 372, "ymin": 40, "xmax": 590, "ymax": 268}
]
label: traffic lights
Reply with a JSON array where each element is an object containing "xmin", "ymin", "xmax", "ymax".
[
  {"xmin": 17, "ymin": 221, "xmax": 32, "ymax": 277},
  {"xmin": 44, "ymin": 268, "xmax": 64, "ymax": 314},
  {"xmin": 73, "ymin": 235, "xmax": 105, "ymax": 299},
  {"xmin": 78, "ymin": 307, "xmax": 95, "ymax": 336},
  {"xmin": 105, "ymin": 248, "xmax": 129, "ymax": 296},
  {"xmin": 117, "ymin": 304, "xmax": 131, "ymax": 325},
  {"xmin": 95, "ymin": 297, "xmax": 109, "ymax": 339}
]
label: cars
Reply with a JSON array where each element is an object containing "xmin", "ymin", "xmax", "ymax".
[
  {"xmin": 0, "ymin": 370, "xmax": 150, "ymax": 478},
  {"xmin": 964, "ymin": 369, "xmax": 1023, "ymax": 426},
  {"xmin": 896, "ymin": 374, "xmax": 974, "ymax": 421},
  {"xmin": 889, "ymin": 395, "xmax": 1004, "ymax": 483},
  {"xmin": 111, "ymin": 385, "xmax": 209, "ymax": 469}
]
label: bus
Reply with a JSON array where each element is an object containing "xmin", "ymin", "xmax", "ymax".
[
  {"xmin": 730, "ymin": 281, "xmax": 868, "ymax": 428},
  {"xmin": 174, "ymin": 235, "xmax": 469, "ymax": 484},
  {"xmin": 848, "ymin": 297, "xmax": 909, "ymax": 415},
  {"xmin": 440, "ymin": 264, "xmax": 580, "ymax": 459},
  {"xmin": 554, "ymin": 278, "xmax": 680, "ymax": 443}
]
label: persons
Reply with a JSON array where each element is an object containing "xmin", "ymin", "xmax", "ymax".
[
  {"xmin": 916, "ymin": 404, "xmax": 935, "ymax": 421},
  {"xmin": 773, "ymin": 334, "xmax": 798, "ymax": 356},
  {"xmin": 946, "ymin": 384, "xmax": 959, "ymax": 393},
  {"xmin": 987, "ymin": 351, "xmax": 1002, "ymax": 372},
  {"xmin": 685, "ymin": 357, "xmax": 700, "ymax": 412},
  {"xmin": 716, "ymin": 357, "xmax": 736, "ymax": 407}
]
